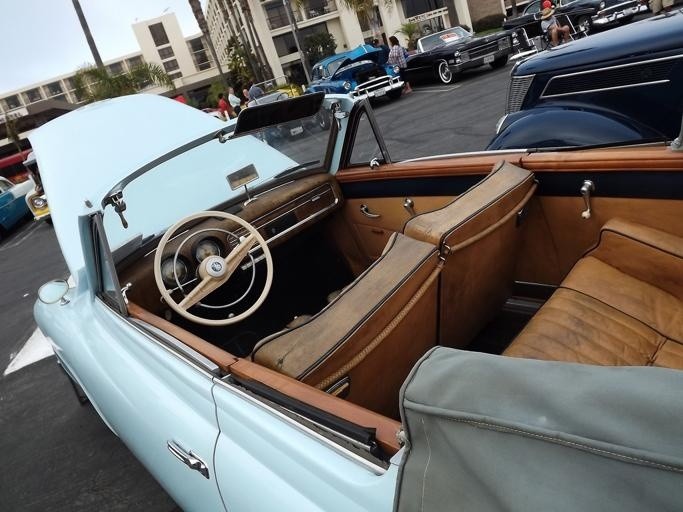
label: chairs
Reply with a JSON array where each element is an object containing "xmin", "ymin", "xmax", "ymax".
[
  {"xmin": 235, "ymin": 229, "xmax": 444, "ymax": 429},
  {"xmin": 324, "ymin": 159, "xmax": 541, "ymax": 354},
  {"xmin": 538, "ymin": 26, "xmax": 563, "ymax": 51}
]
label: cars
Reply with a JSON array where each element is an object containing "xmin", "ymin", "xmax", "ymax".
[{"xmin": 20, "ymin": 86, "xmax": 683, "ymax": 512}]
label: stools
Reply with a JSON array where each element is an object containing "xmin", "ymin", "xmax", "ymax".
[{"xmin": 500, "ymin": 210, "xmax": 681, "ymax": 378}]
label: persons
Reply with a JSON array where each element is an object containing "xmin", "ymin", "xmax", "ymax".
[
  {"xmin": 372, "ymin": 39, "xmax": 389, "ymax": 54},
  {"xmin": 248, "ymin": 81, "xmax": 264, "ymax": 99},
  {"xmin": 217, "ymin": 91, "xmax": 234, "ymax": 121},
  {"xmin": 539, "ymin": 7, "xmax": 570, "ymax": 47},
  {"xmin": 386, "ymin": 34, "xmax": 413, "ymax": 95},
  {"xmin": 241, "ymin": 88, "xmax": 251, "ymax": 107},
  {"xmin": 226, "ymin": 86, "xmax": 242, "ymax": 117}
]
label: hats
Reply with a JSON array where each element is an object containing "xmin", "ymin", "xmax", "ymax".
[{"xmin": 540, "ymin": 7, "xmax": 556, "ymax": 21}]
